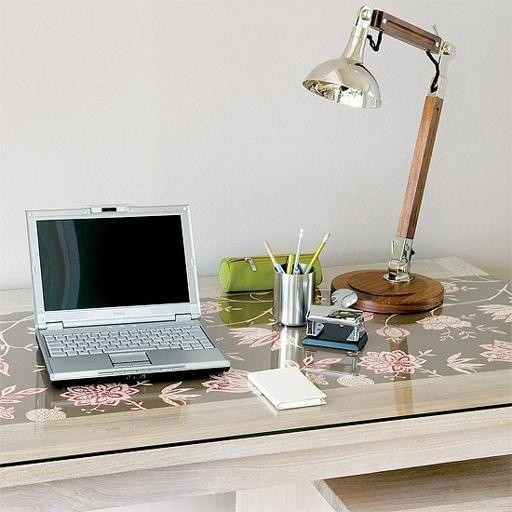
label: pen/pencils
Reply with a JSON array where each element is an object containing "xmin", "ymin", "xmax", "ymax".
[{"xmin": 264, "ymin": 228, "xmax": 331, "ymax": 275}]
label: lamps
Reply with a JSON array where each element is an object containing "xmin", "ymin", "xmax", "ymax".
[{"xmin": 302, "ymin": 4, "xmax": 456, "ymax": 313}]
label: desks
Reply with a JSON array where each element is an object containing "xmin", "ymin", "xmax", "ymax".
[{"xmin": 0, "ymin": 255, "xmax": 512, "ymax": 512}]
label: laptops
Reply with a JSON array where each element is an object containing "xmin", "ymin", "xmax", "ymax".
[{"xmin": 25, "ymin": 205, "xmax": 230, "ymax": 390}]
